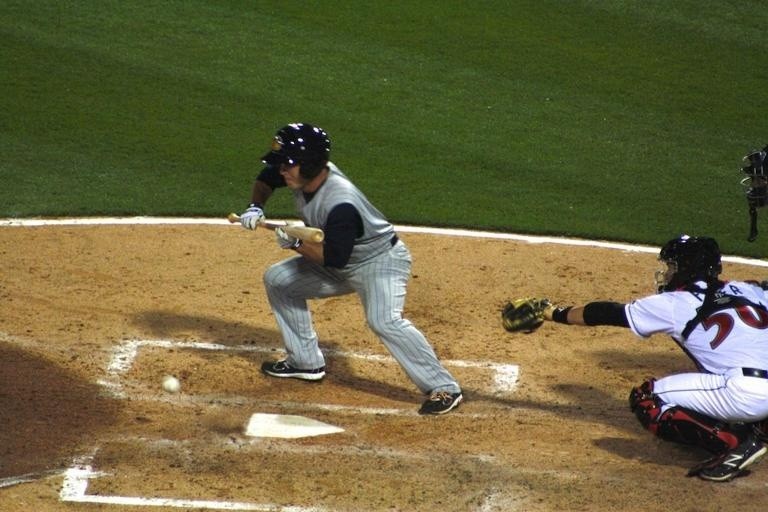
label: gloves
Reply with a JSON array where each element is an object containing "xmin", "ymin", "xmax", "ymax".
[
  {"xmin": 241, "ymin": 202, "xmax": 264, "ymax": 231},
  {"xmin": 274, "ymin": 227, "xmax": 302, "ymax": 251}
]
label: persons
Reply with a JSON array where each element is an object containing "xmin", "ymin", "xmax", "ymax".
[
  {"xmin": 240, "ymin": 123, "xmax": 464, "ymax": 415},
  {"xmin": 740, "ymin": 144, "xmax": 768, "ymax": 242},
  {"xmin": 502, "ymin": 236, "xmax": 768, "ymax": 482}
]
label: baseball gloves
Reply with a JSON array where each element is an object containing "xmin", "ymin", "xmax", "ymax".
[{"xmin": 501, "ymin": 295, "xmax": 552, "ymax": 334}]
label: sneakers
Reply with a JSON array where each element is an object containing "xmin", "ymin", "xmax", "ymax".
[
  {"xmin": 416, "ymin": 388, "xmax": 464, "ymax": 417},
  {"xmin": 260, "ymin": 358, "xmax": 327, "ymax": 382},
  {"xmin": 696, "ymin": 431, "xmax": 767, "ymax": 481}
]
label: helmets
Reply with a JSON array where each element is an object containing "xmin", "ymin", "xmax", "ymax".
[
  {"xmin": 653, "ymin": 234, "xmax": 723, "ymax": 295},
  {"xmin": 740, "ymin": 146, "xmax": 768, "ymax": 209},
  {"xmin": 261, "ymin": 123, "xmax": 331, "ymax": 180}
]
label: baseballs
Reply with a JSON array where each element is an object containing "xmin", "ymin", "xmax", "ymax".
[{"xmin": 163, "ymin": 377, "xmax": 179, "ymax": 391}]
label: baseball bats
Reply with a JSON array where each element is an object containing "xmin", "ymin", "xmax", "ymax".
[{"xmin": 227, "ymin": 212, "xmax": 325, "ymax": 243}]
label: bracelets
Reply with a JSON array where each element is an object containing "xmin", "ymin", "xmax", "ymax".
[
  {"xmin": 552, "ymin": 306, "xmax": 574, "ymax": 325},
  {"xmin": 293, "ymin": 240, "xmax": 302, "ymax": 250}
]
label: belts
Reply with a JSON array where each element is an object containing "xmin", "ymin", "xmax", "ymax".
[{"xmin": 738, "ymin": 363, "xmax": 768, "ymax": 380}]
